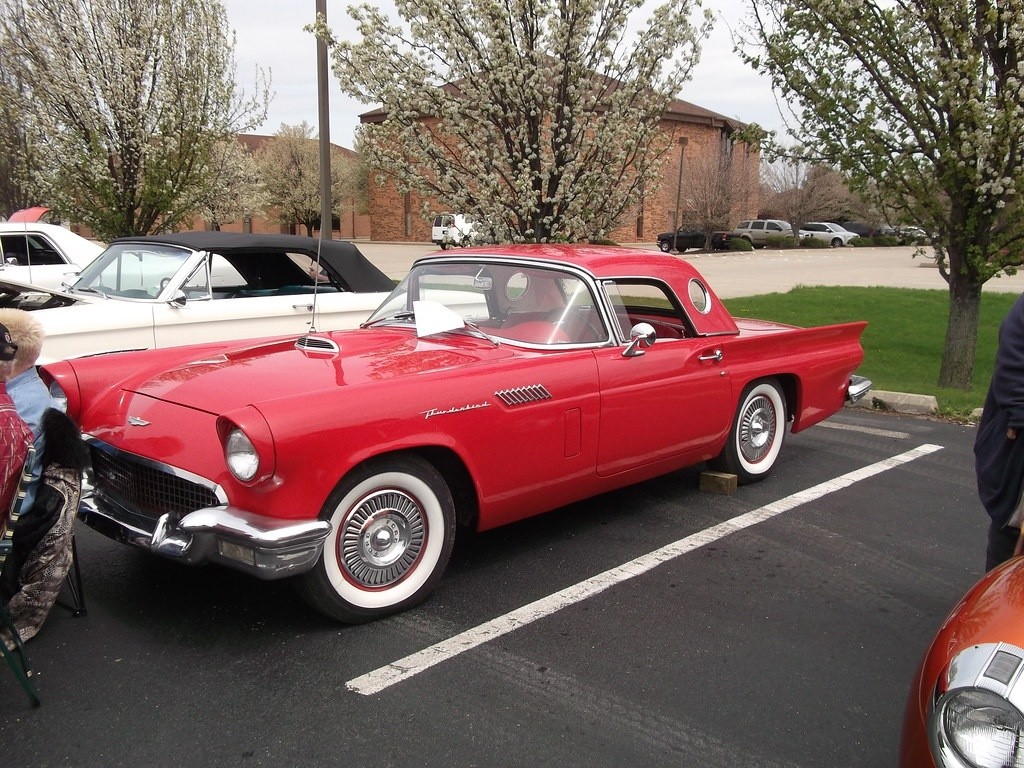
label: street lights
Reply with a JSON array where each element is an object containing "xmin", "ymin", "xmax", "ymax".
[{"xmin": 668, "ymin": 136, "xmax": 690, "ymax": 255}]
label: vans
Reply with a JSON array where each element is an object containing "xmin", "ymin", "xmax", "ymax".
[{"xmin": 432, "ymin": 213, "xmax": 511, "ymax": 251}]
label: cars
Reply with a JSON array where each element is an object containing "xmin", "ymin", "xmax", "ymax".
[
  {"xmin": 0, "ymin": 233, "xmax": 493, "ymax": 382},
  {"xmin": 843, "ymin": 221, "xmax": 940, "ymax": 246},
  {"xmin": 657, "ymin": 221, "xmax": 733, "ymax": 253},
  {"xmin": 798, "ymin": 221, "xmax": 860, "ymax": 248},
  {"xmin": 0, "ymin": 221, "xmax": 248, "ymax": 298}
]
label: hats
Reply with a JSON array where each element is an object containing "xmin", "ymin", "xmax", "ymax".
[{"xmin": 0, "ymin": 321, "xmax": 18, "ymax": 362}]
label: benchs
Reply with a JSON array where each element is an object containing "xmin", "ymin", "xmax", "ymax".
[
  {"xmin": 20, "ymin": 249, "xmax": 60, "ymax": 263},
  {"xmin": 504, "ymin": 311, "xmax": 688, "ymax": 343},
  {"xmin": 229, "ymin": 286, "xmax": 338, "ymax": 298}
]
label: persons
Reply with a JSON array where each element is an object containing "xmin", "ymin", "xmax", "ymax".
[
  {"xmin": 306, "ymin": 266, "xmax": 329, "ymax": 282},
  {"xmin": 974, "ymin": 291, "xmax": 1023, "ymax": 572},
  {"xmin": 0, "ymin": 308, "xmax": 63, "ymax": 542}
]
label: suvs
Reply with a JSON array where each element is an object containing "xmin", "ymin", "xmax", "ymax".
[
  {"xmin": 732, "ymin": 219, "xmax": 814, "ymax": 249},
  {"xmin": 27, "ymin": 244, "xmax": 874, "ymax": 629}
]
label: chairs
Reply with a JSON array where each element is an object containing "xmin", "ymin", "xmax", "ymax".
[{"xmin": 0, "ymin": 408, "xmax": 87, "ymax": 707}]
